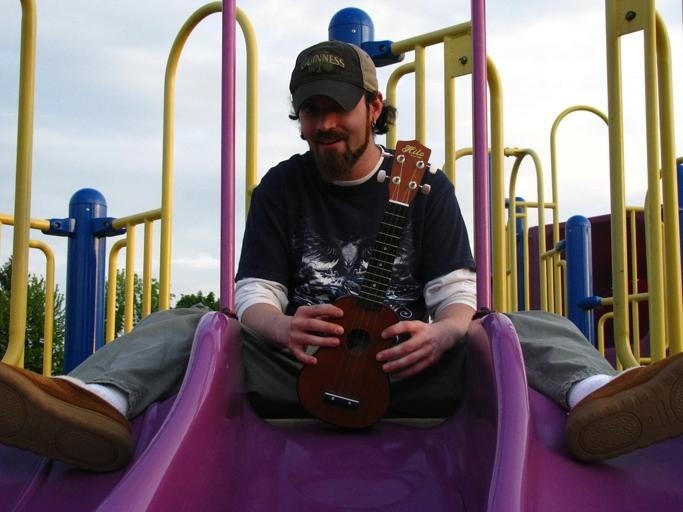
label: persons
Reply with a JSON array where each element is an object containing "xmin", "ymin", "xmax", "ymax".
[{"xmin": 1, "ymin": 41, "xmax": 682, "ymax": 476}]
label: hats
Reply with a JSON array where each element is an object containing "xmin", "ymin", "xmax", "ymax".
[{"xmin": 290, "ymin": 42, "xmax": 378, "ymax": 111}]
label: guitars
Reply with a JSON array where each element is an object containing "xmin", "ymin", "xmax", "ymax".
[{"xmin": 297, "ymin": 141, "xmax": 431, "ymax": 427}]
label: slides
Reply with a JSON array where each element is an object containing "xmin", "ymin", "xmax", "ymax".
[{"xmin": 0, "ymin": 309, "xmax": 682, "ymax": 509}]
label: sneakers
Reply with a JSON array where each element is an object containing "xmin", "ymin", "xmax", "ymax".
[
  {"xmin": 0, "ymin": 361, "xmax": 134, "ymax": 472},
  {"xmin": 565, "ymin": 350, "xmax": 683, "ymax": 462}
]
